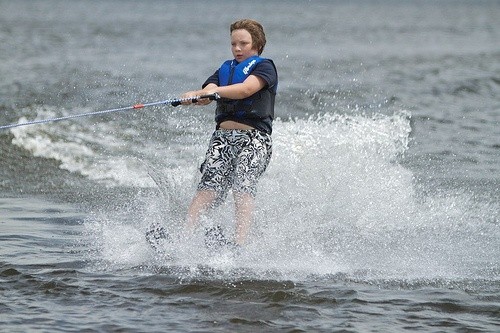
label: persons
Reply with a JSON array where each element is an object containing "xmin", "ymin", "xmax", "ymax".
[{"xmin": 175, "ymin": 19, "xmax": 278, "ymax": 253}]
